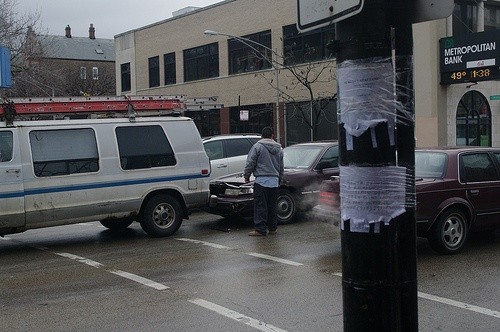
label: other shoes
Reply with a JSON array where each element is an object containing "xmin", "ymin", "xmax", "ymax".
[
  {"xmin": 248, "ymin": 230, "xmax": 267, "ymax": 236},
  {"xmin": 269, "ymin": 230, "xmax": 278, "ymax": 234}
]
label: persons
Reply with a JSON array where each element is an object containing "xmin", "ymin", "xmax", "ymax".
[{"xmin": 244, "ymin": 127, "xmax": 284, "ymax": 235}]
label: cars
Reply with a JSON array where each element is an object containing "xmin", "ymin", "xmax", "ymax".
[
  {"xmin": 212, "ymin": 140, "xmax": 339, "ymax": 224},
  {"xmin": 317, "ymin": 146, "xmax": 497, "ymax": 253},
  {"xmin": 202, "ymin": 134, "xmax": 262, "ymax": 181}
]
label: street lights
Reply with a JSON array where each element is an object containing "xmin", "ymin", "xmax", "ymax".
[{"xmin": 204, "ymin": 29, "xmax": 281, "ymax": 145}]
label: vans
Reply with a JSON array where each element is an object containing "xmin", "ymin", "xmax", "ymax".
[{"xmin": 0, "ymin": 116, "xmax": 212, "ymax": 237}]
